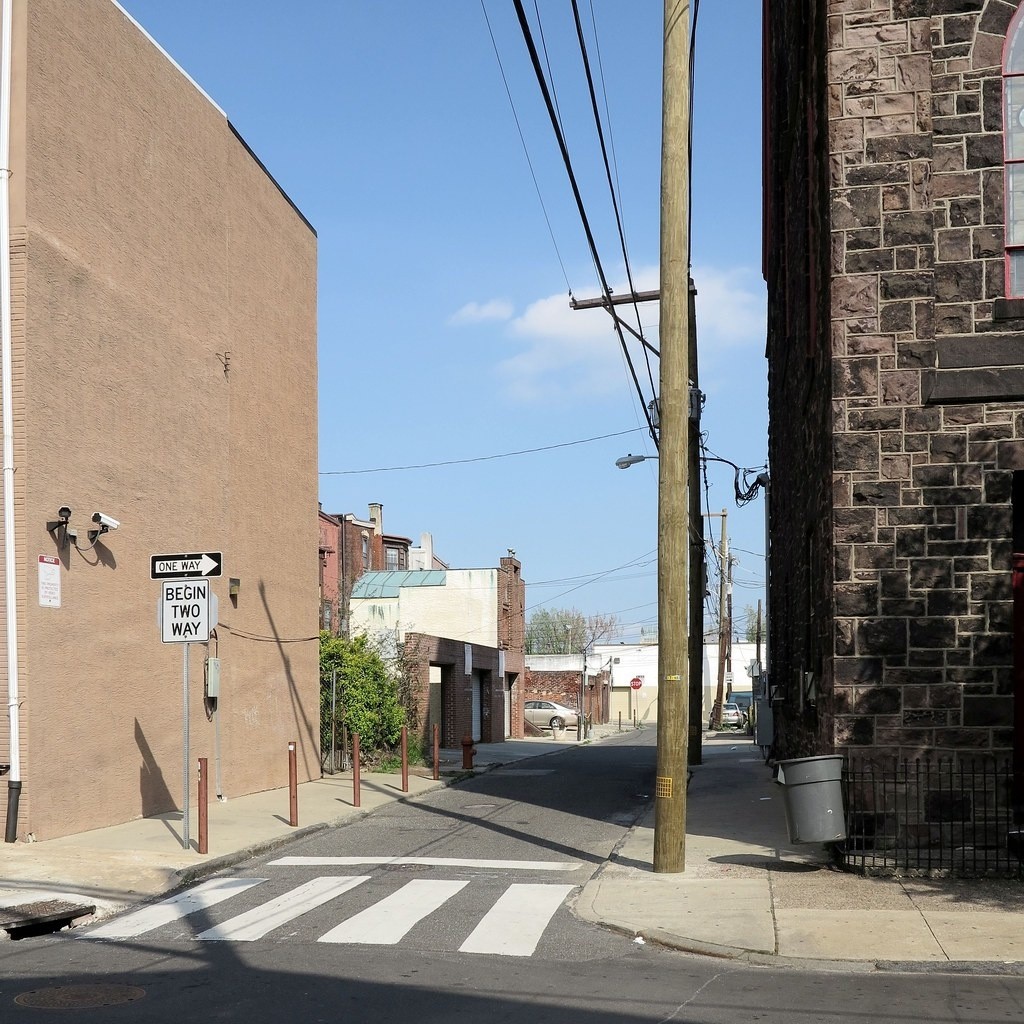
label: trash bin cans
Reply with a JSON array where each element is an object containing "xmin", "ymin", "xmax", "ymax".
[{"xmin": 773, "ymin": 753, "xmax": 848, "ymax": 845}]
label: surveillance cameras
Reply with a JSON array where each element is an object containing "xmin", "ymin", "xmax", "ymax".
[
  {"xmin": 91, "ymin": 512, "xmax": 120, "ymax": 530},
  {"xmin": 58, "ymin": 506, "xmax": 72, "ymax": 517}
]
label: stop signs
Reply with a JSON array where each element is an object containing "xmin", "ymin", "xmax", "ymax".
[{"xmin": 630, "ymin": 678, "xmax": 642, "ymax": 690}]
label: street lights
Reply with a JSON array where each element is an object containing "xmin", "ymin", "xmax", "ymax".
[{"xmin": 616, "ymin": 454, "xmax": 770, "ymax": 766}]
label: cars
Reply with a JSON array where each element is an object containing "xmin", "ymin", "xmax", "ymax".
[
  {"xmin": 719, "ymin": 702, "xmax": 745, "ymax": 728},
  {"xmin": 523, "ymin": 700, "xmax": 588, "ymax": 733}
]
